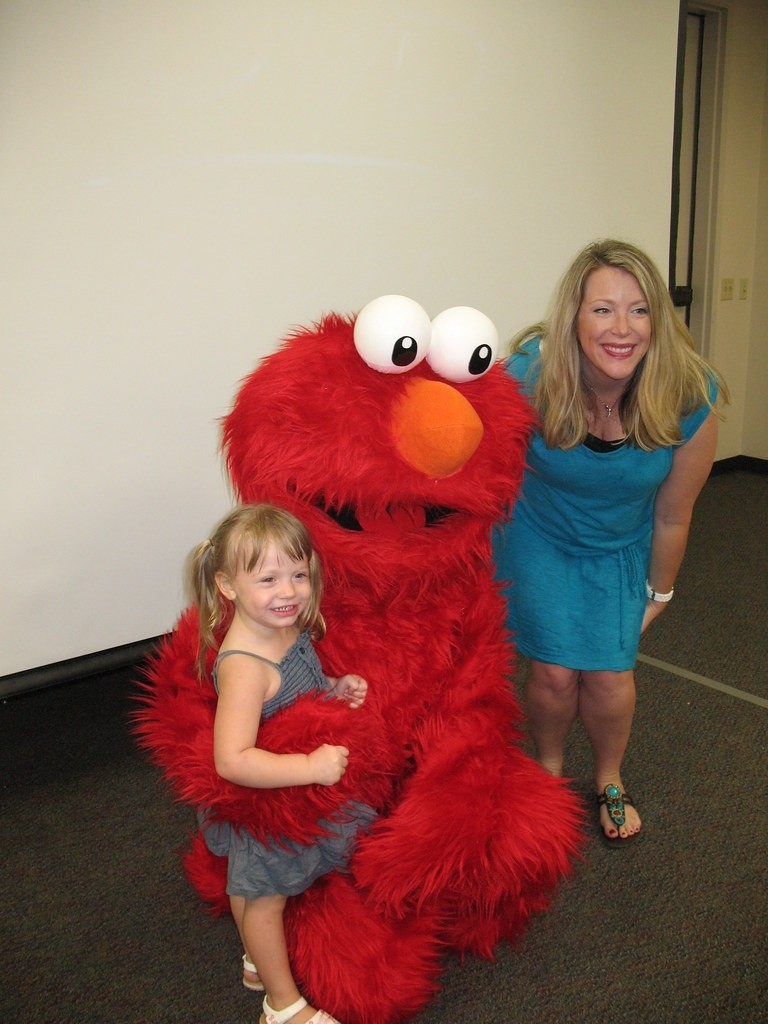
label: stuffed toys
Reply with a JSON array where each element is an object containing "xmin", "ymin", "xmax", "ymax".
[{"xmin": 130, "ymin": 293, "xmax": 591, "ymax": 1024}]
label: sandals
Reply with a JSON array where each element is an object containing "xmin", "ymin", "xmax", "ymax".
[
  {"xmin": 259, "ymin": 994, "xmax": 341, "ymax": 1024},
  {"xmin": 242, "ymin": 955, "xmax": 267, "ymax": 990}
]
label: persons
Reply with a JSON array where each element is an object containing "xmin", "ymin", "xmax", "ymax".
[
  {"xmin": 501, "ymin": 238, "xmax": 732, "ymax": 847},
  {"xmin": 183, "ymin": 503, "xmax": 368, "ymax": 1024}
]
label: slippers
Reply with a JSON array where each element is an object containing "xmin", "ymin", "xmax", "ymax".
[{"xmin": 597, "ymin": 783, "xmax": 640, "ymax": 847}]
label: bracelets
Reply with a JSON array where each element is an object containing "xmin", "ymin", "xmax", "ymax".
[{"xmin": 646, "ymin": 578, "xmax": 674, "ymax": 602}]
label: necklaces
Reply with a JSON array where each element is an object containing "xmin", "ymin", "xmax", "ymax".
[{"xmin": 590, "ymin": 387, "xmax": 618, "ymax": 416}]
